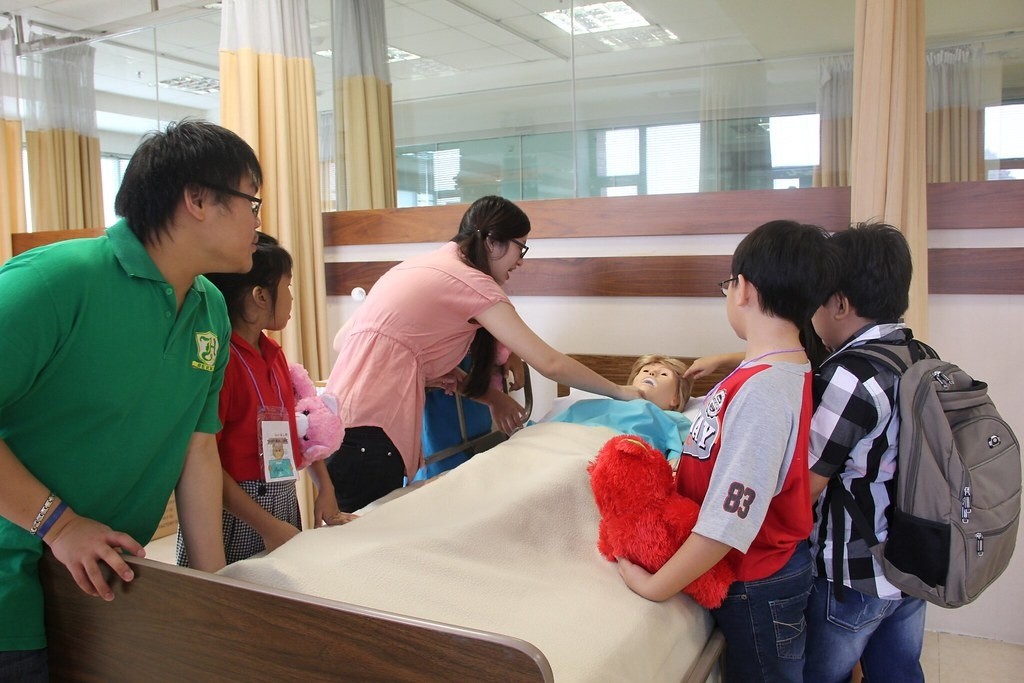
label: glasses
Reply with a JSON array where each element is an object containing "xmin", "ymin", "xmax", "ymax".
[
  {"xmin": 719, "ymin": 278, "xmax": 739, "ymax": 295},
  {"xmin": 510, "ymin": 239, "xmax": 529, "ymax": 258},
  {"xmin": 199, "ymin": 181, "xmax": 262, "ymax": 217}
]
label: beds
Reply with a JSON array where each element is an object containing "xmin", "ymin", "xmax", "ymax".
[{"xmin": 39, "ymin": 354, "xmax": 747, "ymax": 683}]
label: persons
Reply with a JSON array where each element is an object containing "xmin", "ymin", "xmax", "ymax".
[
  {"xmin": 325, "ymin": 193, "xmax": 644, "ymax": 513},
  {"xmin": 616, "ymin": 220, "xmax": 941, "ymax": 683},
  {"xmin": 179, "ymin": 231, "xmax": 359, "ymax": 551},
  {"xmin": 0, "ymin": 115, "xmax": 265, "ymax": 683},
  {"xmin": 547, "ymin": 353, "xmax": 692, "ymax": 476}
]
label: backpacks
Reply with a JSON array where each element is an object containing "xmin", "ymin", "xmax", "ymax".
[{"xmin": 834, "ymin": 339, "xmax": 1021, "ymax": 609}]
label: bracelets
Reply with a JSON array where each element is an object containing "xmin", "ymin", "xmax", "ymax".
[{"xmin": 28, "ymin": 491, "xmax": 70, "ymax": 538}]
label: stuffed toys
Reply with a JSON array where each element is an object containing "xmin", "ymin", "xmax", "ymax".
[
  {"xmin": 284, "ymin": 362, "xmax": 345, "ymax": 470},
  {"xmin": 489, "ymin": 340, "xmax": 514, "ymax": 392},
  {"xmin": 586, "ymin": 433, "xmax": 738, "ymax": 610}
]
